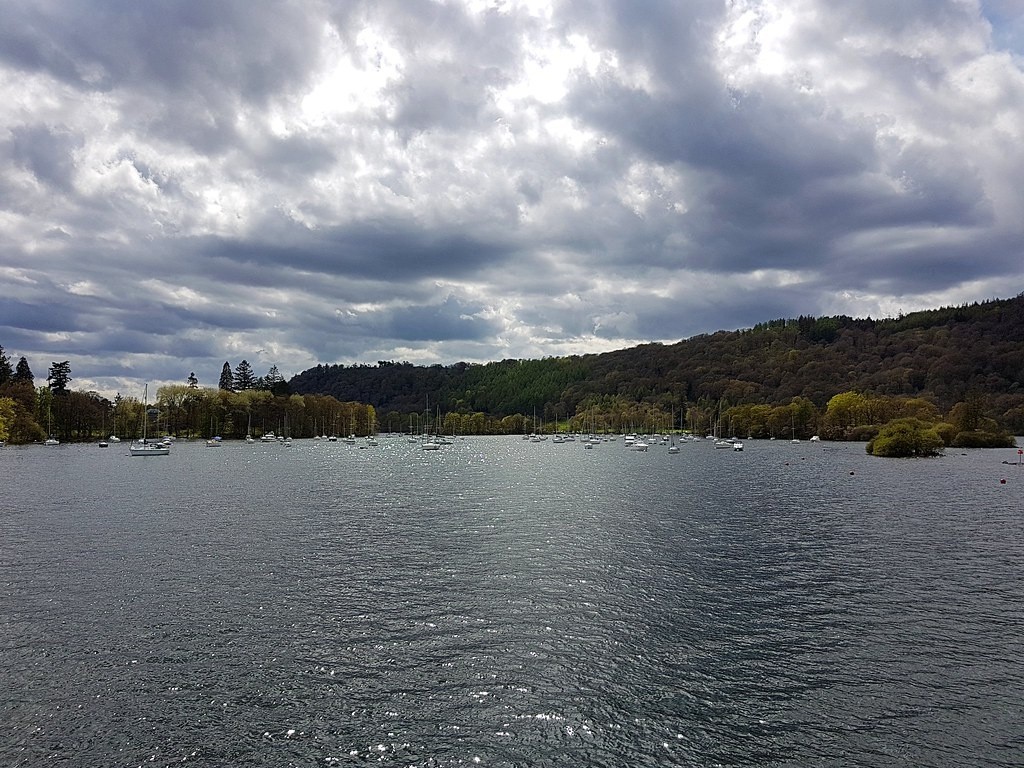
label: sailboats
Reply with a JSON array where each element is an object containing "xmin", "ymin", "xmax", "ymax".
[{"xmin": 129, "ymin": 384, "xmax": 170, "ymax": 456}]
